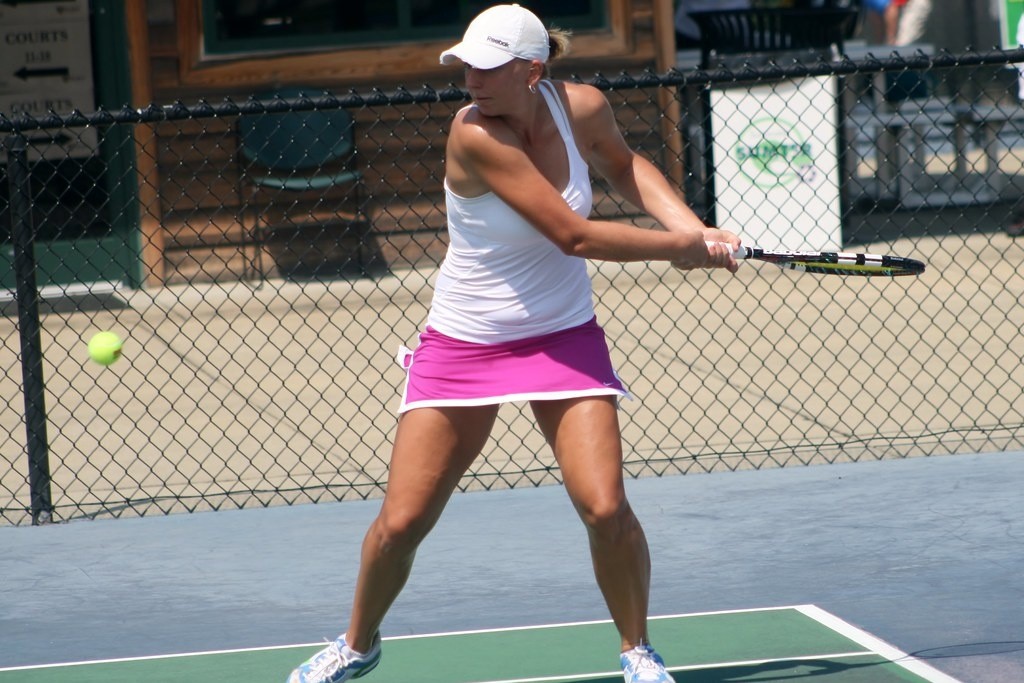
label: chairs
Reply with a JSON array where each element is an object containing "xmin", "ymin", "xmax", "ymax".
[{"xmin": 235, "ymin": 89, "xmax": 367, "ymax": 292}]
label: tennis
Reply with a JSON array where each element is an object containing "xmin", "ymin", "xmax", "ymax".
[{"xmin": 88, "ymin": 332, "xmax": 122, "ymax": 365}]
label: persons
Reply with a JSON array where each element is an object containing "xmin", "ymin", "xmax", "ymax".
[
  {"xmin": 281, "ymin": 3, "xmax": 746, "ymax": 683},
  {"xmin": 871, "ymin": 0, "xmax": 907, "ymax": 46}
]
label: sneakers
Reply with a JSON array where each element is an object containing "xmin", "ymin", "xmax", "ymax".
[
  {"xmin": 286, "ymin": 629, "xmax": 382, "ymax": 683},
  {"xmin": 619, "ymin": 638, "xmax": 676, "ymax": 683}
]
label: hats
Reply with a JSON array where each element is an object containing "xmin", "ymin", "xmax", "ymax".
[{"xmin": 440, "ymin": 4, "xmax": 550, "ymax": 69}]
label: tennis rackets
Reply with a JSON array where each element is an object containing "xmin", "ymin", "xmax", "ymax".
[{"xmin": 706, "ymin": 245, "xmax": 926, "ymax": 276}]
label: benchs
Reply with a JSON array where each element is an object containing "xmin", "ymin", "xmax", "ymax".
[{"xmin": 851, "ymin": 109, "xmax": 1023, "ymax": 209}]
